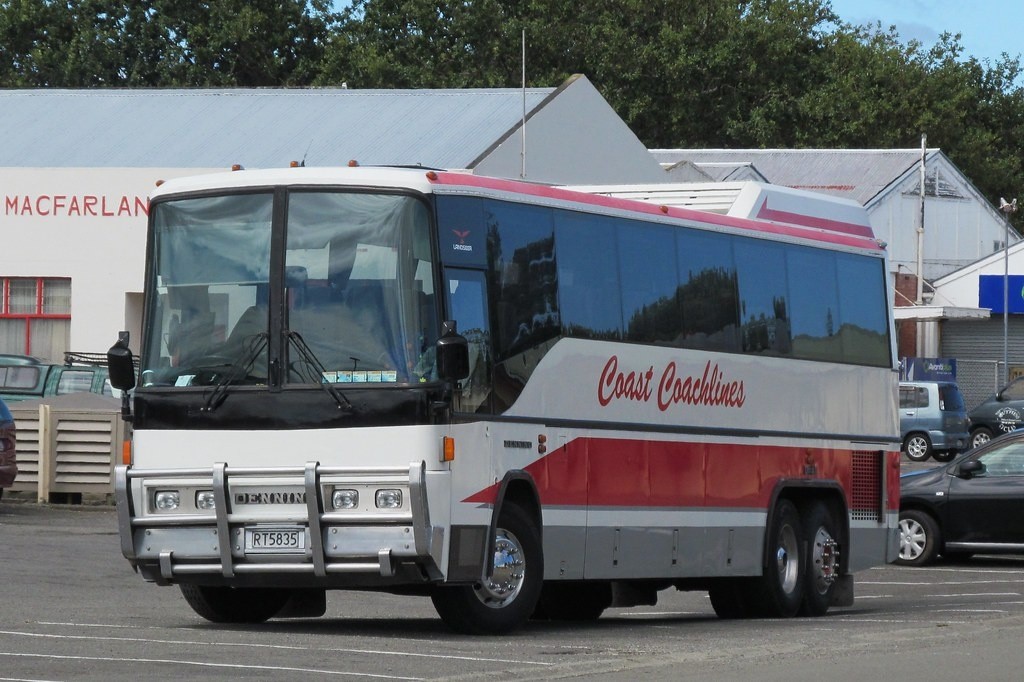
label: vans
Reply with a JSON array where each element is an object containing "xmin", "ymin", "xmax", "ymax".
[
  {"xmin": 899, "ymin": 380, "xmax": 971, "ymax": 461},
  {"xmin": 958, "ymin": 376, "xmax": 1024, "ymax": 453}
]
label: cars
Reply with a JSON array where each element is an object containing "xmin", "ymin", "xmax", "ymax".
[
  {"xmin": 0, "ymin": 398, "xmax": 19, "ymax": 498},
  {"xmin": 895, "ymin": 428, "xmax": 1024, "ymax": 566}
]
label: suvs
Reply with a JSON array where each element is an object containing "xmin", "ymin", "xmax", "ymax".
[
  {"xmin": 0, "ymin": 351, "xmax": 141, "ymax": 402},
  {"xmin": 108, "ymin": 160, "xmax": 900, "ymax": 635}
]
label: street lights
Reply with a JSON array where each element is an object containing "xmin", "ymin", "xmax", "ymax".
[{"xmin": 1000, "ymin": 197, "xmax": 1018, "ymax": 386}]
